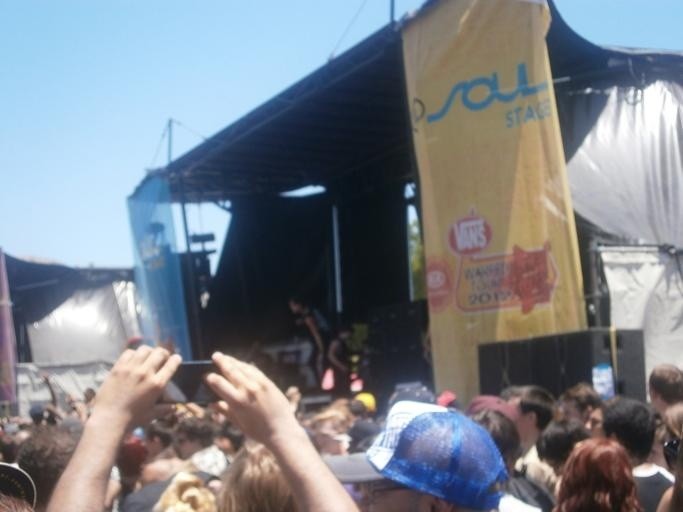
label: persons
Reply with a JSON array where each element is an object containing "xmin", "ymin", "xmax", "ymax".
[{"xmin": 1, "ymin": 294, "xmax": 682, "ymax": 512}]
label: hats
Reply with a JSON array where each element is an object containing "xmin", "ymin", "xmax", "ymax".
[
  {"xmin": 321, "ymin": 401, "xmax": 508, "ymax": 509},
  {"xmin": 467, "ymin": 395, "xmax": 518, "ymax": 429},
  {"xmin": 388, "ymin": 381, "xmax": 436, "ymax": 412}
]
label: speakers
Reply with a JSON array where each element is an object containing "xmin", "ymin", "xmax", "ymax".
[
  {"xmin": 479, "ymin": 328, "xmax": 648, "ymax": 409},
  {"xmin": 360, "ymin": 300, "xmax": 433, "ymax": 415}
]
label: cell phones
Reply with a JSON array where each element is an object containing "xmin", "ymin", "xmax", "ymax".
[{"xmin": 156, "ymin": 361, "xmax": 223, "ymax": 405}]
